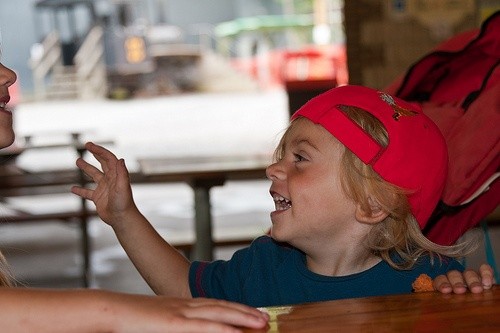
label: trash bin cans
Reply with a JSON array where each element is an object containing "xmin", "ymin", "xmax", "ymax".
[{"xmin": 284, "ymin": 77, "xmax": 339, "ymax": 119}]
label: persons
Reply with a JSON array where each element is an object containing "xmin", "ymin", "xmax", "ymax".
[
  {"xmin": 71, "ymin": 86, "xmax": 496, "ymax": 308},
  {"xmin": 0, "ymin": 63, "xmax": 270, "ymax": 333}
]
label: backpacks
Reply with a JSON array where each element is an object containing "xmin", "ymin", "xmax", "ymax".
[{"xmin": 382, "ymin": 10, "xmax": 499, "ymax": 247}]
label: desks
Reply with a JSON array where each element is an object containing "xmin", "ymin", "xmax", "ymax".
[
  {"xmin": 229, "ymin": 287, "xmax": 500, "ymax": 332},
  {"xmin": 130, "ymin": 141, "xmax": 293, "ymax": 288}
]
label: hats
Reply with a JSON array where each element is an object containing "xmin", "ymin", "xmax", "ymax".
[{"xmin": 290, "ymin": 84, "xmax": 448, "ymax": 230}]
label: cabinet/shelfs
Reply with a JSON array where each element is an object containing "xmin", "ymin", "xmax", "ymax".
[{"xmin": 5, "ymin": 129, "xmax": 122, "ymax": 268}]
